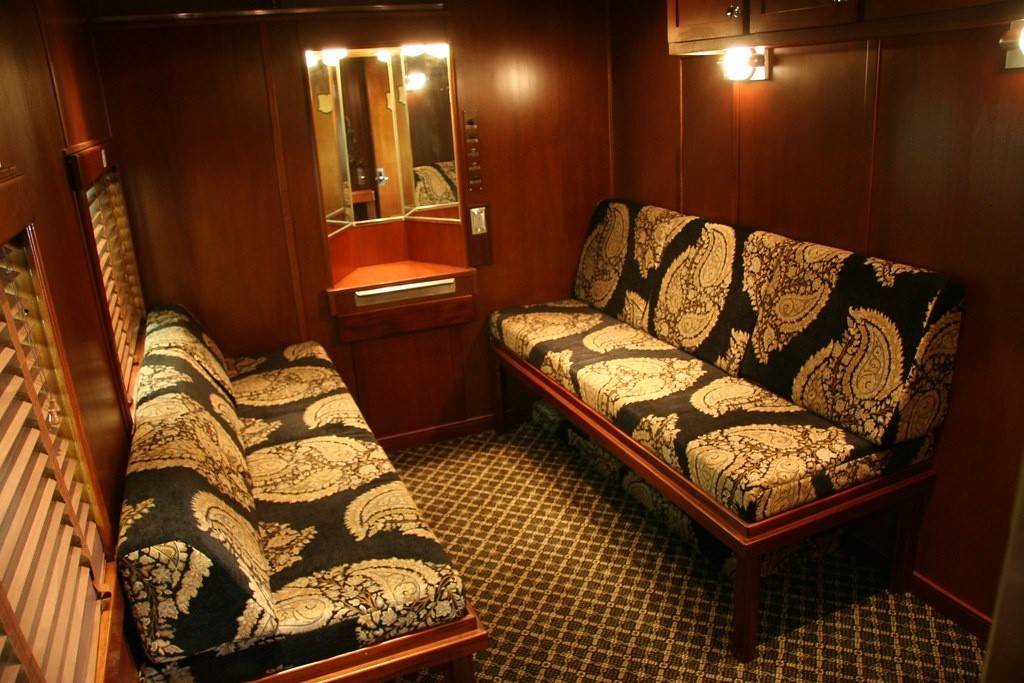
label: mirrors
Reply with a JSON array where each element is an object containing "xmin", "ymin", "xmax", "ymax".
[{"xmin": 307, "ymin": 41, "xmax": 474, "ymax": 294}]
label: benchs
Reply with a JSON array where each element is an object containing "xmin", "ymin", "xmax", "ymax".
[
  {"xmin": 111, "ymin": 299, "xmax": 488, "ymax": 683},
  {"xmin": 486, "ymin": 194, "xmax": 972, "ymax": 663}
]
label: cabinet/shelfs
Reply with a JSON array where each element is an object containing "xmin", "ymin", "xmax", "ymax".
[{"xmin": 664, "ymin": 0, "xmax": 1024, "ymax": 58}]
